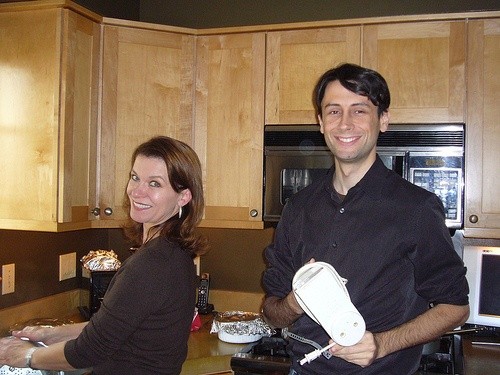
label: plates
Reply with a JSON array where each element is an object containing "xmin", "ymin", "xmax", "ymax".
[{"xmin": 7, "ymin": 317, "xmax": 77, "ymax": 334}]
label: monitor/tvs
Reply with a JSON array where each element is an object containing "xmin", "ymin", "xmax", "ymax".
[{"xmin": 461, "ymin": 245, "xmax": 500, "ymax": 328}]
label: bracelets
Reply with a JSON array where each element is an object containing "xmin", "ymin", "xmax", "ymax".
[{"xmin": 25, "ymin": 345, "xmax": 43, "ymax": 370}]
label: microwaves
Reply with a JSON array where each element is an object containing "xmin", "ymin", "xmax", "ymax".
[{"xmin": 460, "ymin": 245, "xmax": 500, "ymax": 328}]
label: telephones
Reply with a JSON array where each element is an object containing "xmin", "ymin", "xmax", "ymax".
[{"xmin": 196, "ymin": 272, "xmax": 215, "ymax": 316}]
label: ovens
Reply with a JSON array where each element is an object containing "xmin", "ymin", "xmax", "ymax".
[{"xmin": 264, "ymin": 125, "xmax": 464, "ymax": 229}]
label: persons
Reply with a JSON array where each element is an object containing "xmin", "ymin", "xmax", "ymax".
[
  {"xmin": 0, "ymin": 136, "xmax": 209, "ymax": 374},
  {"xmin": 260, "ymin": 60, "xmax": 472, "ymax": 372}
]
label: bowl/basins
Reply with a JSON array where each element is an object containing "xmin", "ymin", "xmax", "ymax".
[{"xmin": 217, "ymin": 313, "xmax": 262, "ymax": 343}]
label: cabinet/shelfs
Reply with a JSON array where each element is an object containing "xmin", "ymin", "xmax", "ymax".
[{"xmin": 0, "ymin": 1, "xmax": 500, "ymax": 241}]
